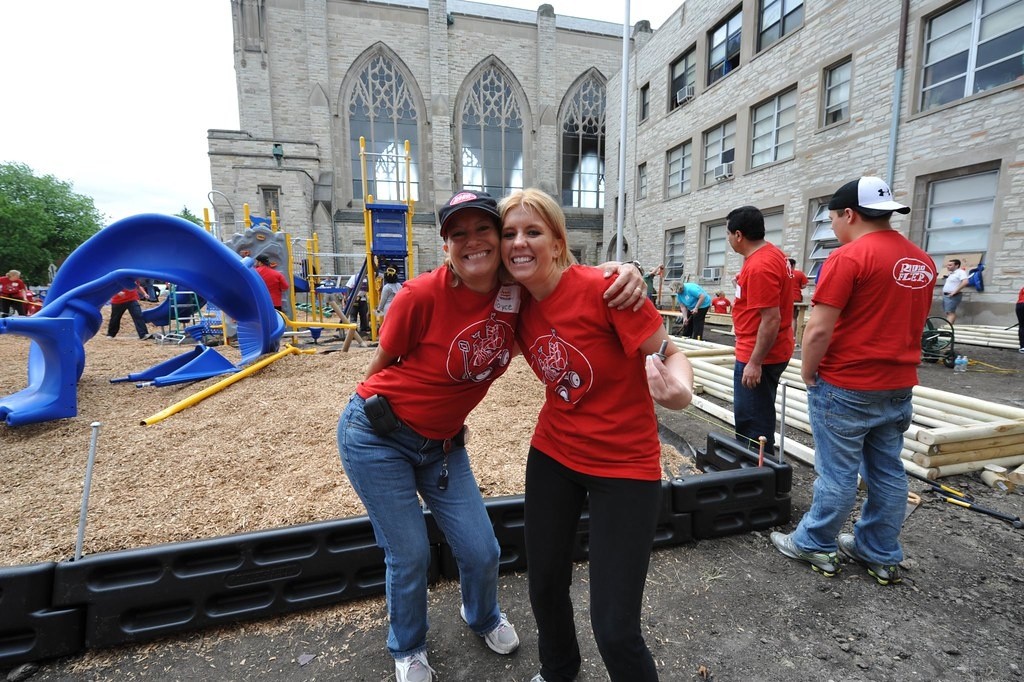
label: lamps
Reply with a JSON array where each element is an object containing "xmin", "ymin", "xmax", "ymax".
[{"xmin": 273, "ymin": 143, "xmax": 284, "ymax": 166}]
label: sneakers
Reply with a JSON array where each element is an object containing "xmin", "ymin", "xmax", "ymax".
[
  {"xmin": 769, "ymin": 532, "xmax": 842, "ymax": 578},
  {"xmin": 393, "ymin": 647, "xmax": 435, "ymax": 682},
  {"xmin": 459, "ymin": 604, "xmax": 520, "ymax": 656},
  {"xmin": 840, "ymin": 532, "xmax": 902, "ymax": 584}
]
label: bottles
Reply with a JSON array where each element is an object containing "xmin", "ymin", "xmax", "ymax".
[
  {"xmin": 960, "ymin": 356, "xmax": 968, "ymax": 373},
  {"xmin": 954, "ymin": 355, "xmax": 961, "ymax": 372}
]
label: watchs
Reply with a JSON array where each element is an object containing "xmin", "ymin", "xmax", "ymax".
[{"xmin": 621, "ymin": 260, "xmax": 644, "ymax": 276}]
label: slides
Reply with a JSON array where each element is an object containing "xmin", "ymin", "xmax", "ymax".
[
  {"xmin": 140, "ymin": 284, "xmax": 208, "ymax": 328},
  {"xmin": 0, "ymin": 213, "xmax": 287, "ymax": 429}
]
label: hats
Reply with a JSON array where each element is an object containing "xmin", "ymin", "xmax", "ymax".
[
  {"xmin": 829, "ymin": 178, "xmax": 912, "ymax": 218},
  {"xmin": 256, "ymin": 255, "xmax": 271, "ymax": 266},
  {"xmin": 439, "ymin": 188, "xmax": 502, "ymax": 237}
]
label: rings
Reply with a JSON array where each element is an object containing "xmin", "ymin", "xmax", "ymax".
[
  {"xmin": 636, "ymin": 287, "xmax": 642, "ymax": 293},
  {"xmin": 642, "ymin": 292, "xmax": 647, "ymax": 295}
]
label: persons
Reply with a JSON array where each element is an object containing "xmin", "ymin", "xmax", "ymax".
[
  {"xmin": 815, "ymin": 249, "xmax": 834, "ymax": 286},
  {"xmin": 789, "ymin": 258, "xmax": 808, "ymax": 339},
  {"xmin": 942, "ymin": 259, "xmax": 968, "ymax": 324},
  {"xmin": 321, "ymin": 274, "xmax": 337, "ymax": 310},
  {"xmin": 0, "ymin": 270, "xmax": 29, "ymax": 318},
  {"xmin": 725, "ymin": 206, "xmax": 795, "ymax": 454},
  {"xmin": 337, "ymin": 190, "xmax": 648, "ymax": 682},
  {"xmin": 255, "ymin": 255, "xmax": 289, "ymax": 310},
  {"xmin": 378, "ymin": 273, "xmax": 402, "ymax": 317},
  {"xmin": 670, "ymin": 281, "xmax": 711, "ymax": 339},
  {"xmin": 107, "ymin": 279, "xmax": 153, "ymax": 340},
  {"xmin": 342, "ymin": 273, "xmax": 371, "ymax": 332},
  {"xmin": 712, "ymin": 292, "xmax": 733, "ymax": 314},
  {"xmin": 643, "ymin": 264, "xmax": 663, "ymax": 300},
  {"xmin": 770, "ymin": 176, "xmax": 937, "ymax": 586},
  {"xmin": 1016, "ymin": 287, "xmax": 1024, "ymax": 353},
  {"xmin": 500, "ymin": 189, "xmax": 693, "ymax": 682}
]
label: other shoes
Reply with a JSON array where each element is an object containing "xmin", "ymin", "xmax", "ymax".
[
  {"xmin": 141, "ymin": 334, "xmax": 153, "ymax": 340},
  {"xmin": 362, "ymin": 327, "xmax": 372, "ymax": 333},
  {"xmin": 1019, "ymin": 348, "xmax": 1024, "ymax": 354}
]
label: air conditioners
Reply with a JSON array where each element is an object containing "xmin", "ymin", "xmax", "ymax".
[
  {"xmin": 702, "ymin": 267, "xmax": 721, "ymax": 281},
  {"xmin": 677, "ymin": 86, "xmax": 693, "ymax": 106},
  {"xmin": 714, "ymin": 163, "xmax": 733, "ymax": 181}
]
